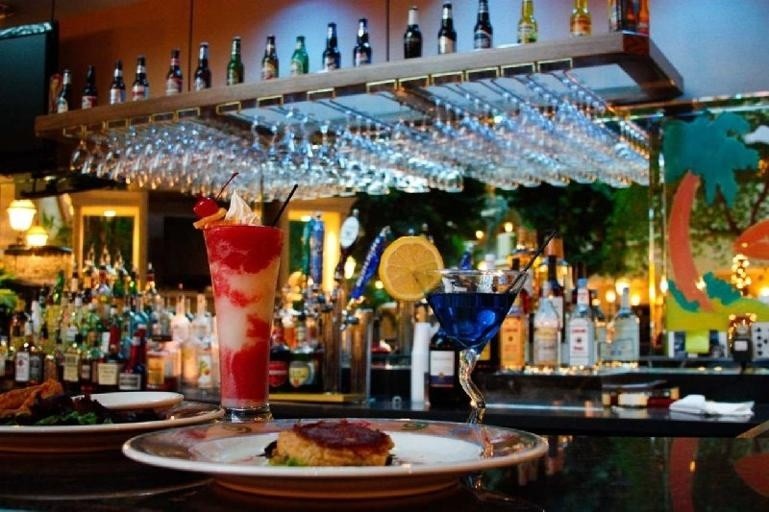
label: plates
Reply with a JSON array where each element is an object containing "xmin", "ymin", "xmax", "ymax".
[
  {"xmin": 123, "ymin": 416, "xmax": 548, "ymax": 499},
  {"xmin": 90, "ymin": 392, "xmax": 183, "ymax": 411},
  {"xmin": 1, "ymin": 399, "xmax": 225, "ymax": 449}
]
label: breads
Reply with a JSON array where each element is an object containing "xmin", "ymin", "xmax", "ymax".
[{"xmin": 272, "ymin": 422, "xmax": 391, "ymax": 467}]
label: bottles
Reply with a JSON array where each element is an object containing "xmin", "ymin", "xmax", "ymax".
[
  {"xmin": 476, "ymin": 0, "xmax": 492, "ymax": 49},
  {"xmin": 454, "ymin": 256, "xmax": 479, "ymax": 293},
  {"xmin": 566, "ymin": 280, "xmax": 598, "ymax": 375},
  {"xmin": 226, "ymin": 37, "xmax": 244, "ymax": 87},
  {"xmin": 404, "ymin": 7, "xmax": 424, "ymax": 59},
  {"xmin": 290, "ymin": 36, "xmax": 307, "ymax": 75},
  {"xmin": 610, "ymin": 289, "xmax": 640, "ymax": 368},
  {"xmin": 322, "ymin": 23, "xmax": 342, "ymax": 73},
  {"xmin": 353, "ymin": 19, "xmax": 371, "ymax": 68},
  {"xmin": 2, "ymin": 247, "xmax": 221, "ymax": 396},
  {"xmin": 165, "ymin": 51, "xmax": 183, "ymax": 97},
  {"xmin": 131, "ymin": 56, "xmax": 149, "ymax": 101},
  {"xmin": 495, "ymin": 273, "xmax": 528, "ymax": 373},
  {"xmin": 533, "ymin": 282, "xmax": 563, "ymax": 368},
  {"xmin": 81, "ymin": 67, "xmax": 97, "ymax": 109},
  {"xmin": 55, "ymin": 70, "xmax": 69, "ymax": 114},
  {"xmin": 519, "ymin": 1, "xmax": 538, "ymax": 44},
  {"xmin": 608, "ymin": 0, "xmax": 650, "ymax": 35},
  {"xmin": 261, "ymin": 35, "xmax": 277, "ymax": 80},
  {"xmin": 193, "ymin": 43, "xmax": 210, "ymax": 91},
  {"xmin": 267, "ymin": 293, "xmax": 351, "ymax": 394},
  {"xmin": 427, "ymin": 323, "xmax": 467, "ymax": 410},
  {"xmin": 108, "ymin": 62, "xmax": 124, "ymax": 104},
  {"xmin": 571, "ymin": 0, "xmax": 591, "ymax": 37},
  {"xmin": 438, "ymin": 2, "xmax": 456, "ymax": 56}
]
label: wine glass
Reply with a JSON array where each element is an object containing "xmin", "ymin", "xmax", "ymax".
[{"xmin": 414, "ymin": 270, "xmax": 530, "ymax": 460}]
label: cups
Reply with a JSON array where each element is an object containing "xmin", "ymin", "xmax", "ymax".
[{"xmin": 202, "ymin": 223, "xmax": 283, "ymax": 423}]
